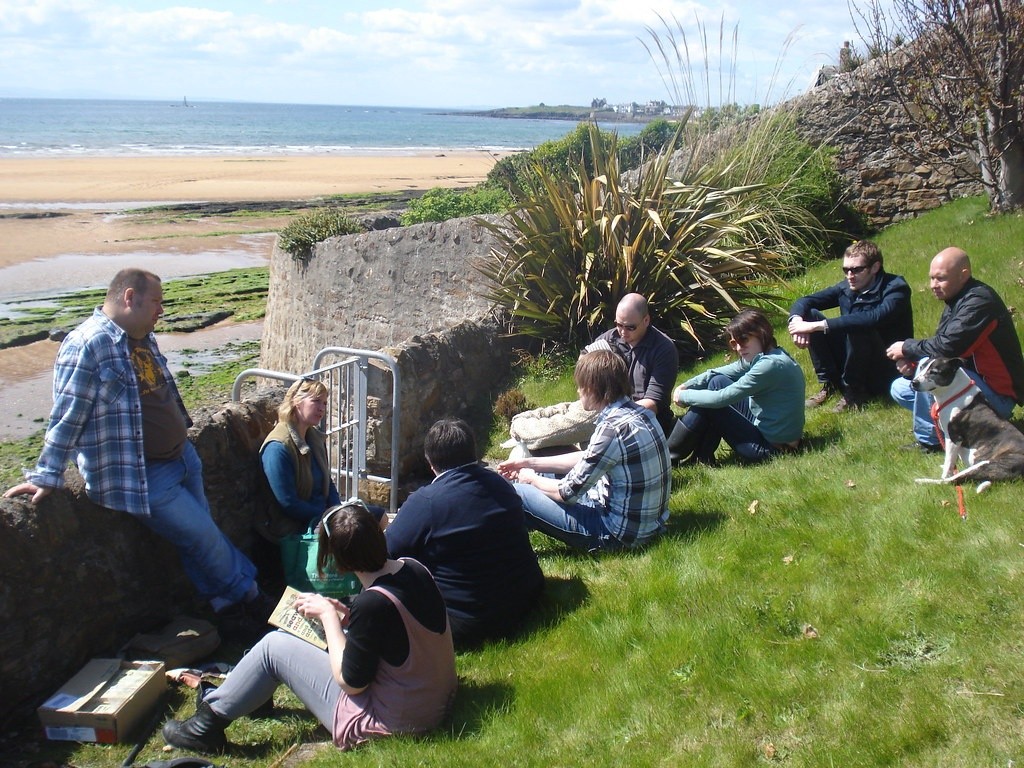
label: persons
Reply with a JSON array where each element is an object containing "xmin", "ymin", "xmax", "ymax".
[
  {"xmin": 383, "ymin": 419, "xmax": 548, "ymax": 649},
  {"xmin": 2, "ymin": 267, "xmax": 278, "ymax": 639},
  {"xmin": 591, "ymin": 293, "xmax": 678, "ymax": 435},
  {"xmin": 161, "ymin": 498, "xmax": 456, "ymax": 754},
  {"xmin": 665, "ymin": 309, "xmax": 805, "ymax": 468},
  {"xmin": 251, "ymin": 378, "xmax": 389, "ymax": 546},
  {"xmin": 885, "ymin": 247, "xmax": 1024, "ymax": 454},
  {"xmin": 788, "ymin": 240, "xmax": 915, "ymax": 413},
  {"xmin": 493, "ymin": 350, "xmax": 672, "ymax": 553}
]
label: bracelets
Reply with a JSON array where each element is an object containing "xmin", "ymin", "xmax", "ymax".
[{"xmin": 822, "ymin": 319, "xmax": 830, "ymax": 335}]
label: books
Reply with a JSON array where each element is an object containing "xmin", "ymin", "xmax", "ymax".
[{"xmin": 268, "ymin": 586, "xmax": 347, "ymax": 651}]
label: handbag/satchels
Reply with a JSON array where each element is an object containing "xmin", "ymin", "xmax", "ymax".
[
  {"xmin": 116, "ymin": 616, "xmax": 222, "ymax": 669},
  {"xmin": 280, "ymin": 515, "xmax": 362, "ymax": 599}
]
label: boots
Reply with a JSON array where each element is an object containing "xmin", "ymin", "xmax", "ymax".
[
  {"xmin": 680, "ymin": 428, "xmax": 721, "ymax": 468},
  {"xmin": 244, "ymin": 649, "xmax": 274, "ymax": 716},
  {"xmin": 666, "ymin": 417, "xmax": 698, "ymax": 470},
  {"xmin": 161, "ymin": 681, "xmax": 233, "ymax": 757}
]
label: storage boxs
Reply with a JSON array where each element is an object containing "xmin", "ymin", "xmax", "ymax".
[{"xmin": 37, "ymin": 658, "xmax": 169, "ymax": 745}]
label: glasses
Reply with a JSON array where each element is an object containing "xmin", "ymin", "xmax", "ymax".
[
  {"xmin": 843, "ymin": 264, "xmax": 871, "ymax": 274},
  {"xmin": 614, "ymin": 314, "xmax": 646, "ymax": 331},
  {"xmin": 291, "ymin": 376, "xmax": 315, "ymax": 399},
  {"xmin": 323, "ymin": 499, "xmax": 370, "ymax": 538},
  {"xmin": 729, "ymin": 332, "xmax": 756, "ymax": 350}
]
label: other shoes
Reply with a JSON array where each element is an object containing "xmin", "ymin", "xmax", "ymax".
[
  {"xmin": 832, "ymin": 397, "xmax": 864, "ymax": 414},
  {"xmin": 805, "ymin": 388, "xmax": 837, "ymax": 408},
  {"xmin": 241, "ymin": 568, "xmax": 280, "ymax": 628},
  {"xmin": 901, "ymin": 441, "xmax": 941, "ymax": 454}
]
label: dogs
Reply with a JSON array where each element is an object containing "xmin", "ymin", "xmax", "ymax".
[{"xmin": 909, "ymin": 355, "xmax": 1024, "ymax": 494}]
